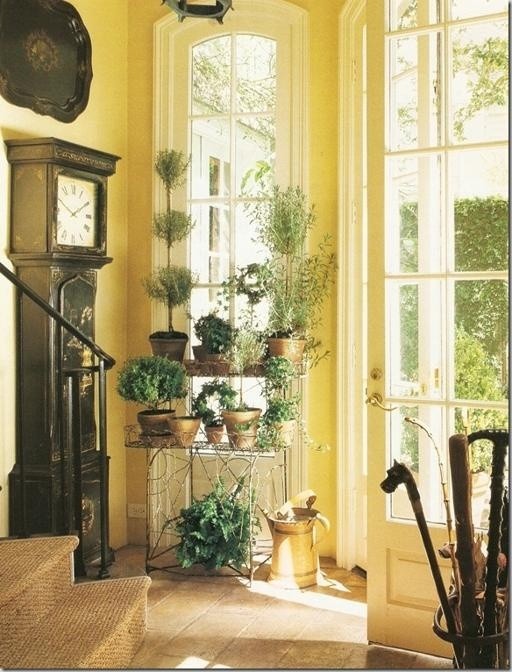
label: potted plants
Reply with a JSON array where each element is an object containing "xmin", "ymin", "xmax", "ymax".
[
  {"xmin": 119, "ymin": 354, "xmax": 190, "ymax": 445},
  {"xmin": 164, "ymin": 415, "xmax": 200, "ymax": 448},
  {"xmin": 190, "ymin": 375, "xmax": 237, "ymax": 446},
  {"xmin": 138, "ymin": 144, "xmax": 203, "ymax": 365},
  {"xmin": 212, "ymin": 324, "xmax": 262, "ymax": 451},
  {"xmin": 182, "ymin": 304, "xmax": 234, "ymax": 375},
  {"xmin": 223, "ymin": 258, "xmax": 275, "ymax": 374},
  {"xmin": 224, "ymin": 140, "xmax": 339, "ymax": 370},
  {"xmin": 256, "ymin": 354, "xmax": 332, "ymax": 458}
]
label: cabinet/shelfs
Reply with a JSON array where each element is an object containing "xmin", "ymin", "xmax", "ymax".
[{"xmin": 134, "ymin": 359, "xmax": 306, "ymax": 590}]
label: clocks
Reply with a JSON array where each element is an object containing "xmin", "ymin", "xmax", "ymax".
[{"xmin": 0, "ymin": 135, "xmax": 119, "ymax": 581}]
